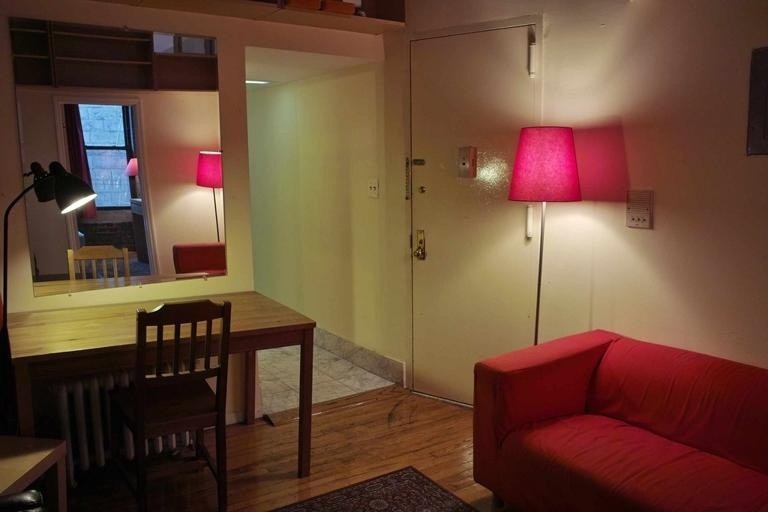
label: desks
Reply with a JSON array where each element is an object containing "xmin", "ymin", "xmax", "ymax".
[
  {"xmin": 0, "ymin": 289, "xmax": 321, "ymax": 482},
  {"xmin": 129, "ymin": 195, "xmax": 144, "ymax": 264},
  {"xmin": 32, "ymin": 271, "xmax": 177, "ymax": 297}
]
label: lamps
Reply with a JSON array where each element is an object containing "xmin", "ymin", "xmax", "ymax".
[
  {"xmin": 195, "ymin": 149, "xmax": 222, "ymax": 243},
  {"xmin": 508, "ymin": 125, "xmax": 582, "ymax": 345},
  {"xmin": 22, "ymin": 161, "xmax": 53, "ymax": 203},
  {"xmin": 0, "ymin": 157, "xmax": 98, "ymax": 328},
  {"xmin": 122, "ymin": 158, "xmax": 138, "ymax": 179}
]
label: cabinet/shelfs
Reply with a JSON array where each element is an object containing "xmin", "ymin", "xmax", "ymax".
[{"xmin": 9, "ymin": 15, "xmax": 219, "ymax": 91}]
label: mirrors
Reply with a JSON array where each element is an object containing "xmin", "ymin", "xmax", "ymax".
[{"xmin": 6, "ymin": 8, "xmax": 232, "ymax": 298}]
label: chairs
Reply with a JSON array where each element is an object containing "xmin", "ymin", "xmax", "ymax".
[
  {"xmin": 172, "ymin": 242, "xmax": 227, "ymax": 277},
  {"xmin": 105, "ymin": 299, "xmax": 234, "ymax": 512},
  {"xmin": 62, "ymin": 242, "xmax": 130, "ymax": 277}
]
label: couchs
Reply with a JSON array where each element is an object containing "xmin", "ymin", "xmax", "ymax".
[{"xmin": 472, "ymin": 326, "xmax": 767, "ymax": 510}]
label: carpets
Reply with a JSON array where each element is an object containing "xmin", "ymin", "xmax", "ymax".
[{"xmin": 267, "ymin": 463, "xmax": 479, "ymax": 511}]
